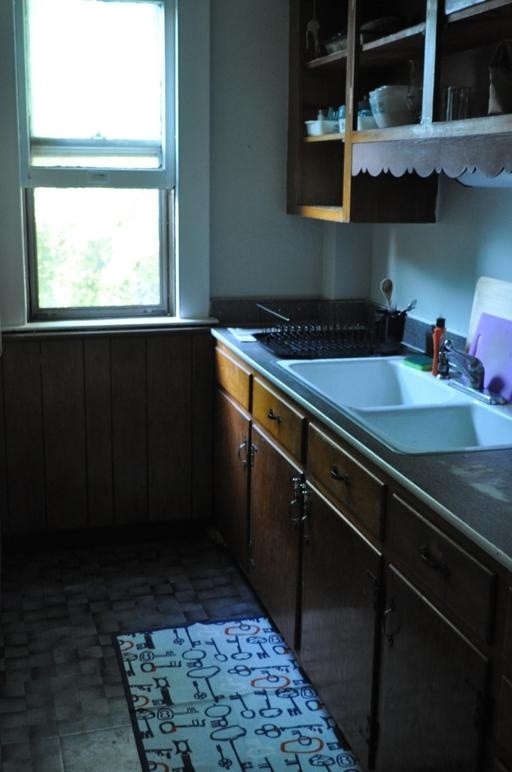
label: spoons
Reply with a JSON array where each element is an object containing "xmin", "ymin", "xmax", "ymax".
[
  {"xmin": 400, "ymin": 299, "xmax": 418, "ymax": 315},
  {"xmin": 380, "ymin": 278, "xmax": 395, "ymax": 313}
]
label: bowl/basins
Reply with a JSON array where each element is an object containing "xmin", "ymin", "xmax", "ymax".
[{"xmin": 369, "ymin": 84, "xmax": 421, "ymax": 127}]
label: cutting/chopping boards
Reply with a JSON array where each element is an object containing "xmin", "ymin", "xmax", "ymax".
[
  {"xmin": 462, "ymin": 312, "xmax": 512, "ymax": 402},
  {"xmin": 464, "ymin": 276, "xmax": 512, "ymax": 355}
]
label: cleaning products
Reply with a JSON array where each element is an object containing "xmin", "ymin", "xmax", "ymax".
[{"xmin": 430, "ymin": 317, "xmax": 448, "ymax": 377}]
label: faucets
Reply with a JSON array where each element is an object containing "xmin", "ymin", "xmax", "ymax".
[{"xmin": 439, "ymin": 338, "xmax": 503, "ymax": 404}]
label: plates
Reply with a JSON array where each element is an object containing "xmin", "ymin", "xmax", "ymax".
[{"xmin": 251, "ymin": 331, "xmax": 383, "ymax": 358}]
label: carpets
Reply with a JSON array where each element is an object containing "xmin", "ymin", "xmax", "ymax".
[{"xmin": 114, "ymin": 617, "xmax": 357, "ymax": 771}]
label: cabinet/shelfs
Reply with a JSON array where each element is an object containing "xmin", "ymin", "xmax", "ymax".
[
  {"xmin": 388, "ymin": 470, "xmax": 512, "ymax": 771},
  {"xmin": 287, "ymin": 1, "xmax": 512, "ymax": 224},
  {"xmin": 249, "ymin": 376, "xmax": 386, "ymax": 771},
  {"xmin": 210, "ymin": 344, "xmax": 252, "ymax": 571}
]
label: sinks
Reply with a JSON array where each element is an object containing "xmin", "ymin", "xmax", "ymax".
[
  {"xmin": 345, "ymin": 401, "xmax": 512, "ymax": 455},
  {"xmin": 278, "ymin": 355, "xmax": 486, "ymax": 406}
]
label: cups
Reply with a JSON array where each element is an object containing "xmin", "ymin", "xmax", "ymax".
[
  {"xmin": 442, "ymin": 87, "xmax": 456, "ymax": 120},
  {"xmin": 456, "ymin": 86, "xmax": 471, "ymax": 121}
]
